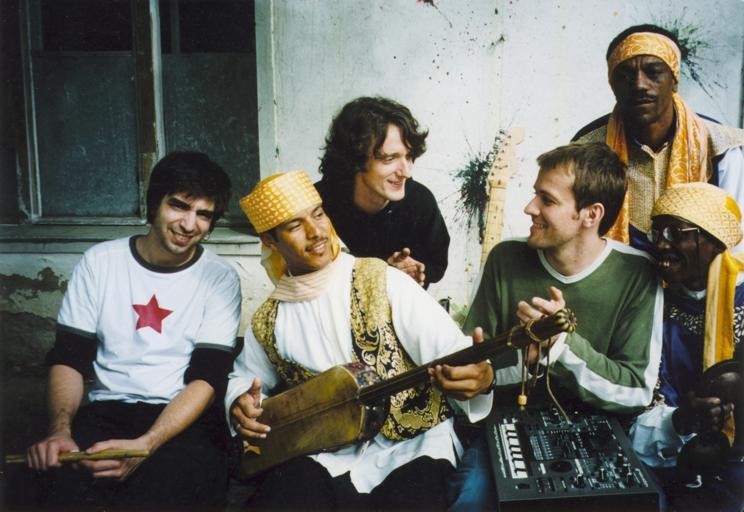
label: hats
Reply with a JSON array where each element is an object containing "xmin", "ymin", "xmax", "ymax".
[
  {"xmin": 652, "ymin": 180, "xmax": 744, "ymax": 248},
  {"xmin": 237, "ymin": 170, "xmax": 322, "ymax": 236}
]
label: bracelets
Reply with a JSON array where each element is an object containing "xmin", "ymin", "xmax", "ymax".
[{"xmin": 482, "ymin": 364, "xmax": 497, "ymax": 394}]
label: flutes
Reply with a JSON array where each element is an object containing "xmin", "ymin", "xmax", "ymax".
[{"xmin": 4, "ymin": 448, "xmax": 151, "ymax": 466}]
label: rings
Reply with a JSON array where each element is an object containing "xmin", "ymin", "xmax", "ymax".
[{"xmin": 235, "ymin": 423, "xmax": 241, "ymax": 430}]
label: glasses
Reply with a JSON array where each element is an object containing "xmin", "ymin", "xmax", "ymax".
[{"xmin": 644, "ymin": 226, "xmax": 698, "ymax": 243}]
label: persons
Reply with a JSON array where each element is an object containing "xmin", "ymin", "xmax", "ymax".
[
  {"xmin": 313, "ymin": 97, "xmax": 450, "ymax": 290},
  {"xmin": 570, "ymin": 26, "xmax": 744, "ymax": 256},
  {"xmin": 446, "ymin": 142, "xmax": 664, "ymax": 512},
  {"xmin": 225, "ymin": 170, "xmax": 497, "ymax": 512},
  {"xmin": 628, "ymin": 181, "xmax": 744, "ymax": 512},
  {"xmin": 26, "ymin": 151, "xmax": 241, "ymax": 512}
]
